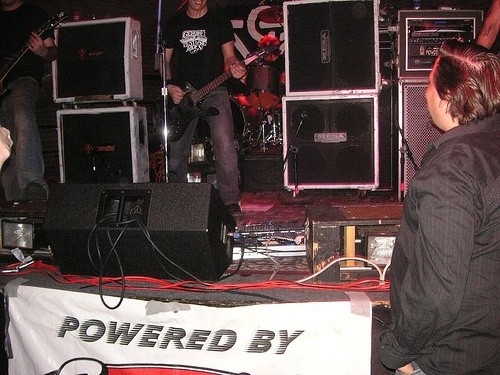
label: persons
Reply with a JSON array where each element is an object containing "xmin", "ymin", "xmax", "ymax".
[
  {"xmin": 380, "ymin": 39, "xmax": 500, "ymax": 375},
  {"xmin": 160, "ymin": 0, "xmax": 248, "ymax": 217},
  {"xmin": 0, "ymin": 0, "xmax": 60, "ymax": 202}
]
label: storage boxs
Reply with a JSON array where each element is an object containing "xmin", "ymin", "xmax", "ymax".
[{"xmin": 303, "ymin": 204, "xmax": 404, "ymax": 283}]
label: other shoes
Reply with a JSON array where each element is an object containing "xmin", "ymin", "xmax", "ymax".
[
  {"xmin": 24, "ymin": 183, "xmax": 47, "ymax": 204},
  {"xmin": 227, "ymin": 205, "xmax": 243, "ymax": 219}
]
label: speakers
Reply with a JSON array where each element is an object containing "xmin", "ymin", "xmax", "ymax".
[
  {"xmin": 51, "ymin": 16, "xmax": 153, "ymax": 185},
  {"xmin": 44, "ymin": 181, "xmax": 237, "ymax": 283},
  {"xmin": 281, "ymin": 0, "xmax": 447, "ymax": 202}
]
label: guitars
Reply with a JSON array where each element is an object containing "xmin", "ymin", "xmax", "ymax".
[
  {"xmin": 151, "ymin": 41, "xmax": 278, "ymax": 143},
  {"xmin": 0, "ymin": 11, "xmax": 69, "ymax": 96}
]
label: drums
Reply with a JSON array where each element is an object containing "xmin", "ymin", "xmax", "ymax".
[
  {"xmin": 176, "ymin": 97, "xmax": 251, "ymax": 159},
  {"xmin": 247, "ymin": 65, "xmax": 278, "ymax": 107}
]
